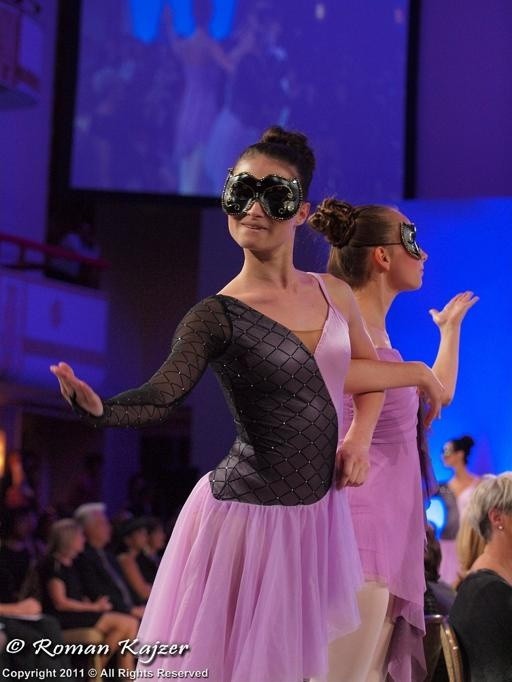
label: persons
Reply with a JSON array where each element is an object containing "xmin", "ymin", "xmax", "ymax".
[
  {"xmin": 48, "ymin": 124, "xmax": 388, "ymax": 682},
  {"xmin": 1, "ymin": 465, "xmax": 176, "ymax": 682},
  {"xmin": 307, "ymin": 194, "xmax": 482, "ymax": 682},
  {"xmin": 423, "ymin": 435, "xmax": 512, "ymax": 681}
]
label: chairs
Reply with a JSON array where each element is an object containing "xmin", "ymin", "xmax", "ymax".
[
  {"xmin": 417, "ymin": 614, "xmax": 447, "ymax": 680},
  {"xmin": 438, "ymin": 619, "xmax": 464, "ymax": 681}
]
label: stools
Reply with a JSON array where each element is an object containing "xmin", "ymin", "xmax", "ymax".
[{"xmin": 61, "ymin": 625, "xmax": 105, "ymax": 679}]
